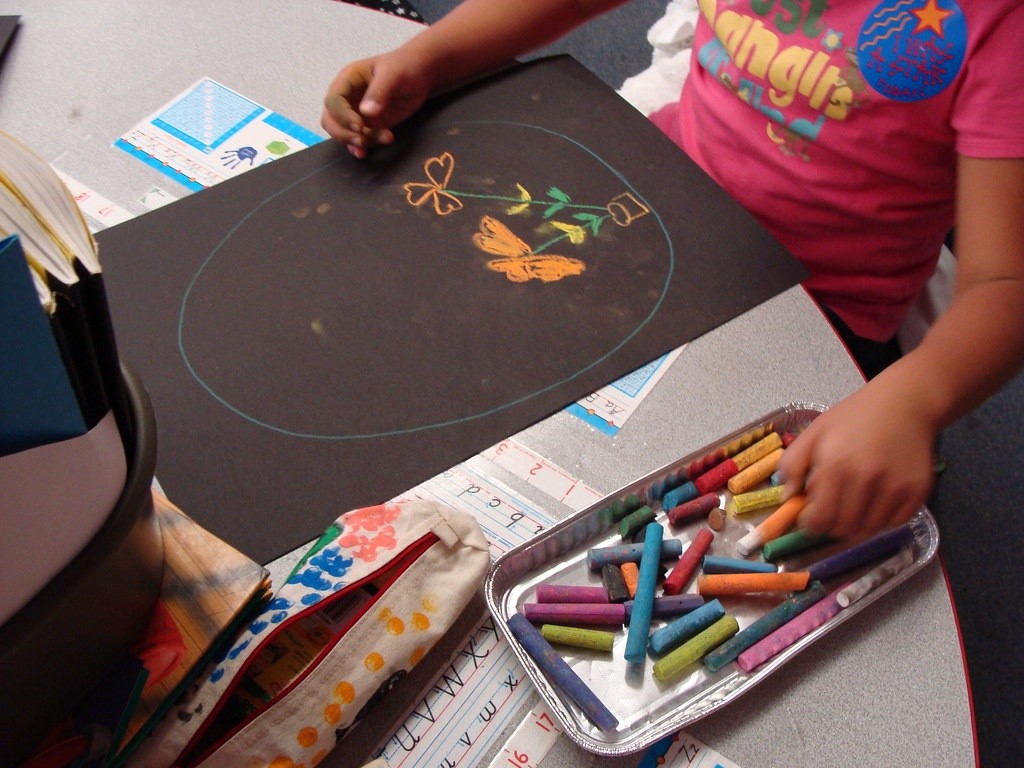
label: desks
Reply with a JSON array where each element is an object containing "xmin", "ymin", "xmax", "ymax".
[{"xmin": 0, "ymin": 0, "xmax": 978, "ymax": 768}]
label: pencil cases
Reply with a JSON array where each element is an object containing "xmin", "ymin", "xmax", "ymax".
[{"xmin": 118, "ymin": 497, "xmax": 491, "ymax": 768}]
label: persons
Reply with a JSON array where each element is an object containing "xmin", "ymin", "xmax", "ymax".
[{"xmin": 319, "ymin": 0, "xmax": 1024, "ymax": 543}]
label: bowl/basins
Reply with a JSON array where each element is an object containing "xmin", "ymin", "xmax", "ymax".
[{"xmin": 0, "ymin": 306, "xmax": 168, "ymax": 768}]
